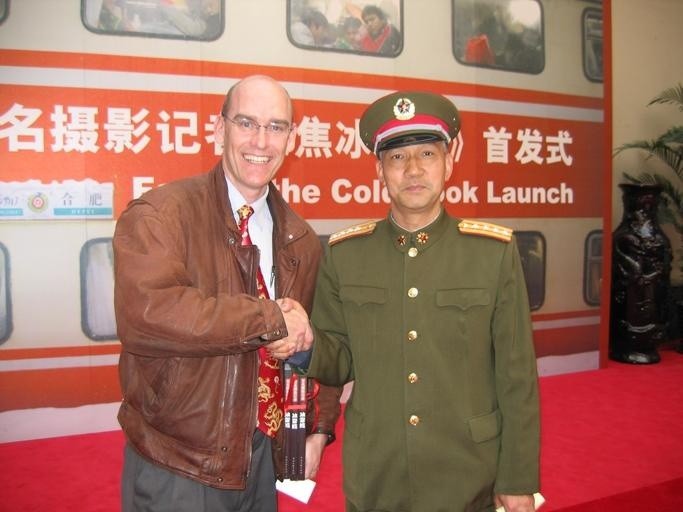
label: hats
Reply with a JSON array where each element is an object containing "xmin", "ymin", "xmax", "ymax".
[{"xmin": 359, "ymin": 91, "xmax": 460, "ymax": 161}]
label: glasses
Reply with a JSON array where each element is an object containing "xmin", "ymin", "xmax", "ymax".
[{"xmin": 222, "ymin": 113, "xmax": 290, "ymax": 139}]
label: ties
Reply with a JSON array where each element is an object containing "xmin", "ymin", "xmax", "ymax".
[{"xmin": 236, "ymin": 204, "xmax": 285, "ymax": 439}]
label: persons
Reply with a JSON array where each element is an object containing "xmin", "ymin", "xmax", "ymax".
[
  {"xmin": 289, "ymin": 4, "xmax": 402, "ymax": 55},
  {"xmin": 460, "ymin": 0, "xmax": 542, "ymax": 71},
  {"xmin": 96, "ymin": 0, "xmax": 222, "ymax": 36},
  {"xmin": 111, "ymin": 72, "xmax": 345, "ymax": 512},
  {"xmin": 260, "ymin": 88, "xmax": 544, "ymax": 511}
]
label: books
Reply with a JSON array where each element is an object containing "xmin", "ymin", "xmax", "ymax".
[{"xmin": 278, "ymin": 361, "xmax": 307, "ymax": 483}]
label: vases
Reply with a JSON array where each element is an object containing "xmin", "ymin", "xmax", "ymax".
[{"xmin": 609, "ymin": 182, "xmax": 671, "ymax": 364}]
